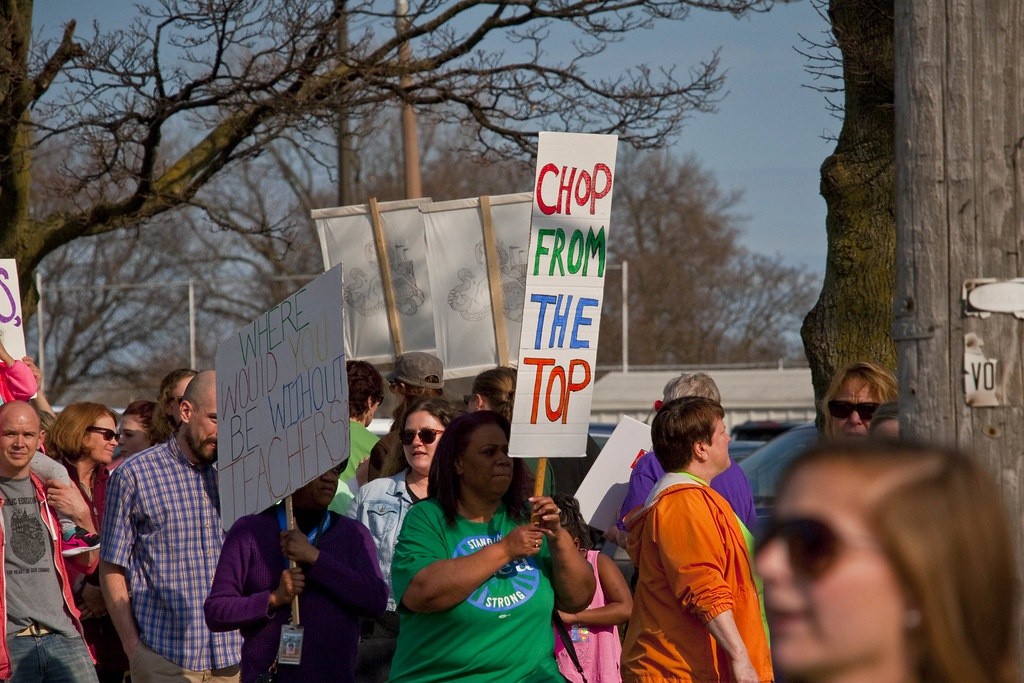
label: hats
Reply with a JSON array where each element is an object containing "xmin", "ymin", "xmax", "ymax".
[{"xmin": 385, "ymin": 352, "xmax": 444, "ymax": 390}]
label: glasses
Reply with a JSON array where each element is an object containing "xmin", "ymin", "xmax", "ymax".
[
  {"xmin": 398, "ymin": 428, "xmax": 445, "ymax": 445},
  {"xmin": 827, "ymin": 399, "xmax": 880, "ymax": 420},
  {"xmin": 750, "ymin": 517, "xmax": 889, "ymax": 571},
  {"xmin": 86, "ymin": 425, "xmax": 121, "ymax": 442}
]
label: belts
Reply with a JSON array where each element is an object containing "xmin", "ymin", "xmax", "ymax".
[{"xmin": 16, "ymin": 623, "xmax": 52, "ymax": 636}]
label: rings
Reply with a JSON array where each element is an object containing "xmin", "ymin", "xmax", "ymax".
[
  {"xmin": 50, "ymin": 494, "xmax": 52, "ymax": 500},
  {"xmin": 535, "ymin": 539, "xmax": 538, "ymax": 547}
]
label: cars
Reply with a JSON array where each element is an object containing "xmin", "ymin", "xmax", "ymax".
[{"xmin": 727, "ymin": 420, "xmax": 821, "ymax": 542}]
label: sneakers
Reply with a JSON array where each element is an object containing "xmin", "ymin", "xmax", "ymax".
[{"xmin": 61, "ymin": 526, "xmax": 103, "ymax": 556}]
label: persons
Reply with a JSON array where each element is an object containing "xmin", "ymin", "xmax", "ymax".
[
  {"xmin": 752, "ymin": 437, "xmax": 1023, "ymax": 683},
  {"xmin": 0, "ymin": 342, "xmax": 632, "ymax": 683},
  {"xmin": 614, "ymin": 372, "xmax": 773, "ymax": 683},
  {"xmin": 821, "ymin": 361, "xmax": 900, "ymax": 441},
  {"xmin": 869, "ymin": 400, "xmax": 900, "ymax": 440}
]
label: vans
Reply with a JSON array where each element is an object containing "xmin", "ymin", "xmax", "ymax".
[{"xmin": 591, "ymin": 367, "xmax": 821, "ymax": 445}]
